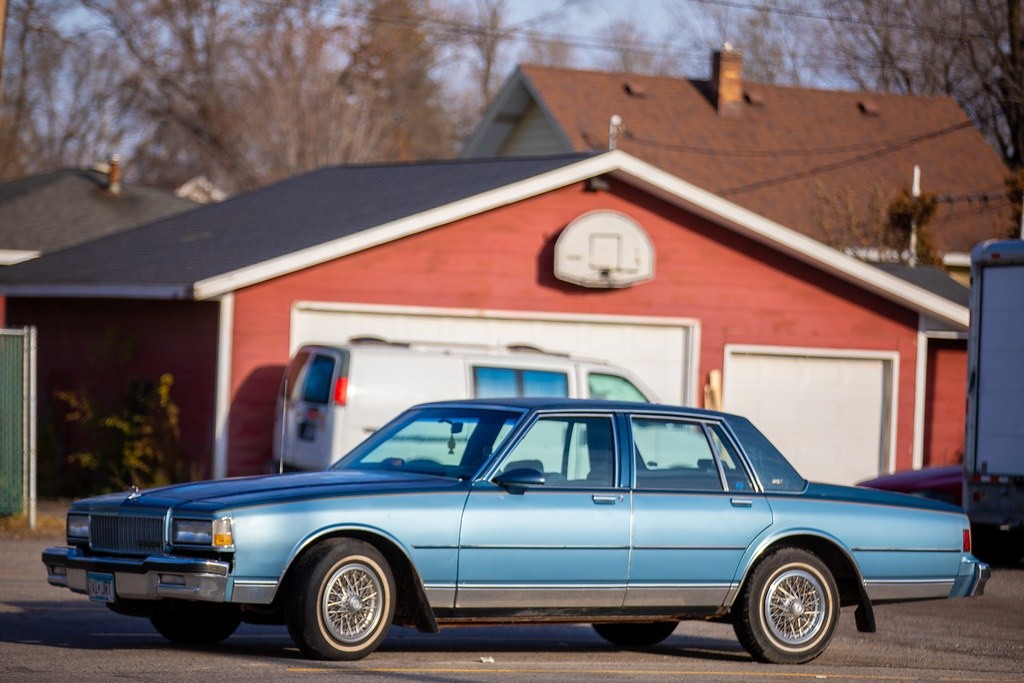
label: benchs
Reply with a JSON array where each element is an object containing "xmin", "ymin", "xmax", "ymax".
[{"xmin": 570, "ymin": 457, "xmax": 745, "ymax": 491}]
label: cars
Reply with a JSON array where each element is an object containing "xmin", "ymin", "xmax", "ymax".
[
  {"xmin": 855, "ymin": 465, "xmax": 968, "ymax": 516},
  {"xmin": 43, "ymin": 394, "xmax": 991, "ymax": 662}
]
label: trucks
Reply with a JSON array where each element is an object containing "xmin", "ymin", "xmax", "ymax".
[{"xmin": 960, "ymin": 234, "xmax": 1023, "ymax": 566}]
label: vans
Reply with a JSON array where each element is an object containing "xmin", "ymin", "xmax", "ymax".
[{"xmin": 271, "ymin": 335, "xmax": 655, "ymax": 472}]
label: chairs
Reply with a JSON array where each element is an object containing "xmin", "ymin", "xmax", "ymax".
[
  {"xmin": 560, "ymin": 457, "xmax": 613, "ymax": 489},
  {"xmin": 502, "ymin": 457, "xmax": 564, "ymax": 487}
]
